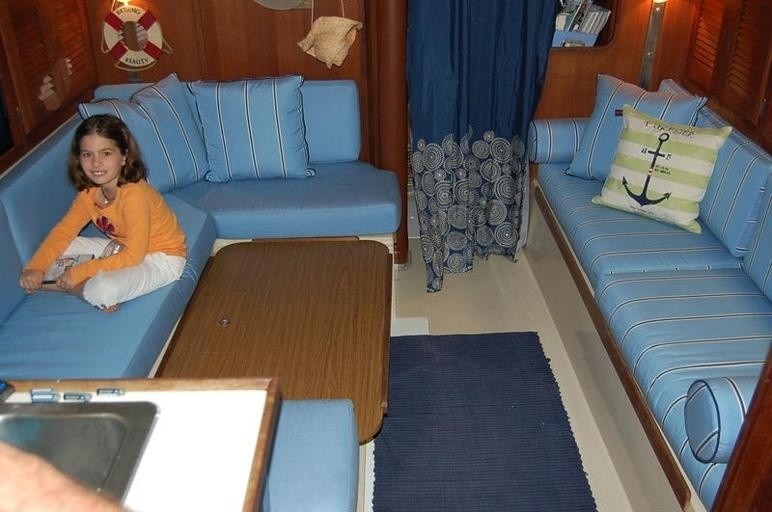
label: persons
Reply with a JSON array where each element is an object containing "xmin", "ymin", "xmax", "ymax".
[
  {"xmin": 1, "ymin": 435, "xmax": 135, "ymax": 511},
  {"xmin": 16, "ymin": 112, "xmax": 188, "ymax": 312}
]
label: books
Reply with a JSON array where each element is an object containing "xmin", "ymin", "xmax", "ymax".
[{"xmin": 554, "ymin": 0, "xmax": 612, "ymax": 36}]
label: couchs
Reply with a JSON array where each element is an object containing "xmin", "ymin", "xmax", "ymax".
[
  {"xmin": 528, "ymin": 77, "xmax": 771, "ymax": 512},
  {"xmin": 1, "ymin": 77, "xmax": 398, "ymax": 512}
]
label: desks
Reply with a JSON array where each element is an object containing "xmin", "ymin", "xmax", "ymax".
[{"xmin": 0, "ymin": 378, "xmax": 280, "ymax": 508}]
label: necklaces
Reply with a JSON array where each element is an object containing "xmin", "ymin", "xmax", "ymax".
[{"xmin": 101, "ymin": 187, "xmax": 114, "ymax": 205}]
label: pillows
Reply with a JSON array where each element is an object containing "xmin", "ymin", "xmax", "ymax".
[
  {"xmin": 77, "ymin": 73, "xmax": 317, "ymax": 192},
  {"xmin": 564, "ymin": 73, "xmax": 735, "ymax": 237}
]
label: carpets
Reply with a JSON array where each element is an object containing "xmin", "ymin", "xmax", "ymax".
[{"xmin": 367, "ymin": 330, "xmax": 599, "ymax": 512}]
